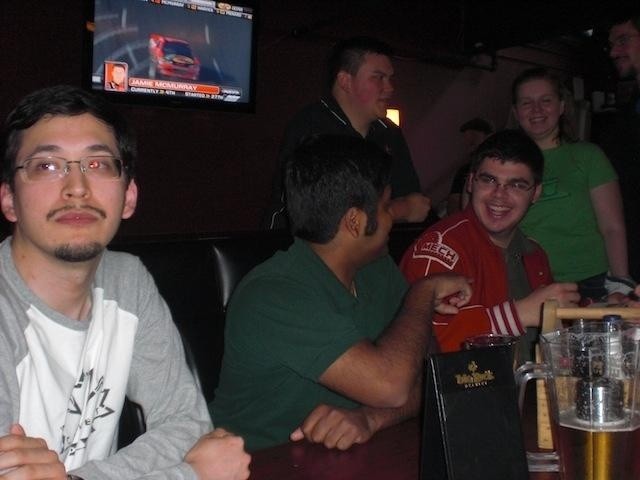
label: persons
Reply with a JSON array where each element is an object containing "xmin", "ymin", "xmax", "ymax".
[
  {"xmin": 208, "ymin": 129, "xmax": 474, "ymax": 450},
  {"xmin": 459, "ymin": 68, "xmax": 629, "ymax": 325},
  {"xmin": 399, "ymin": 129, "xmax": 582, "ymax": 353},
  {"xmin": 259, "ymin": 33, "xmax": 432, "ymax": 264},
  {"xmin": 0, "ymin": 82, "xmax": 252, "ymax": 480},
  {"xmin": 425, "ymin": 118, "xmax": 494, "ymax": 211},
  {"xmin": 592, "ymin": 11, "xmax": 640, "ymax": 301}
]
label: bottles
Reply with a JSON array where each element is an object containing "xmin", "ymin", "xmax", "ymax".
[
  {"xmin": 596, "ymin": 315, "xmax": 627, "ymax": 377},
  {"xmin": 569, "ymin": 316, "xmax": 595, "ymax": 373},
  {"xmin": 573, "ymin": 376, "xmax": 627, "ymax": 479}
]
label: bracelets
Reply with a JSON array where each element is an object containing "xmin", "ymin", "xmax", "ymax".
[{"xmin": 612, "ymin": 273, "xmax": 628, "ymax": 280}]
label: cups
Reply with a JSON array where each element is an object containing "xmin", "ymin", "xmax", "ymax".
[
  {"xmin": 515, "ymin": 328, "xmax": 640, "ymax": 479},
  {"xmin": 461, "ymin": 333, "xmax": 518, "ymax": 349}
]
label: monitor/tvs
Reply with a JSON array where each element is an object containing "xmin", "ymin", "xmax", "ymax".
[{"xmin": 85, "ymin": 0, "xmax": 258, "ymax": 114}]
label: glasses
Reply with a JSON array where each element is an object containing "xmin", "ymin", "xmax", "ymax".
[
  {"xmin": 13, "ymin": 153, "xmax": 127, "ymax": 183},
  {"xmin": 603, "ymin": 34, "xmax": 638, "ymax": 51},
  {"xmin": 471, "ymin": 172, "xmax": 538, "ymax": 195}
]
